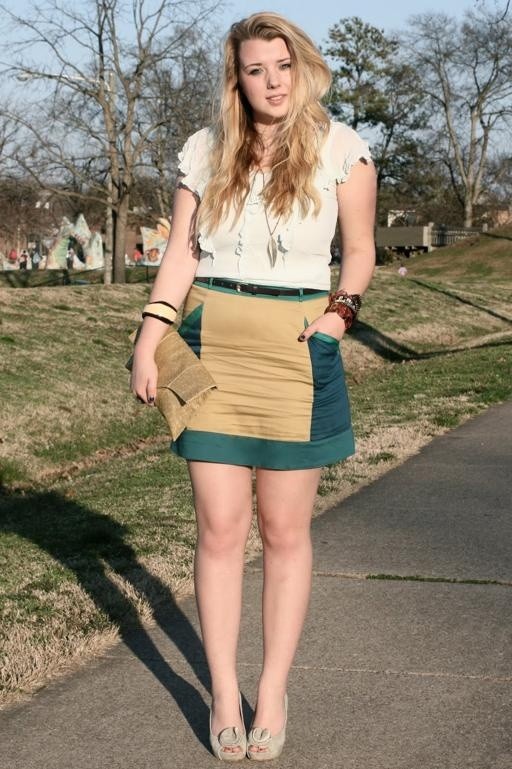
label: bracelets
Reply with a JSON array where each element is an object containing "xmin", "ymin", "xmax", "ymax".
[
  {"xmin": 324, "ymin": 290, "xmax": 361, "ymax": 329},
  {"xmin": 142, "ymin": 300, "xmax": 178, "ymax": 325}
]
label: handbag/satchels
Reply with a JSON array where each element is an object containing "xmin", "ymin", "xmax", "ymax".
[{"xmin": 126, "ymin": 323, "xmax": 216, "ymax": 443}]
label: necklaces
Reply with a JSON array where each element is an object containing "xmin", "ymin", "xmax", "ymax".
[{"xmin": 256, "ymin": 160, "xmax": 283, "ymax": 266}]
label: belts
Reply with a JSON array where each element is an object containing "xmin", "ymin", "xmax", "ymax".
[{"xmin": 195, "ymin": 276, "xmax": 321, "ymax": 296}]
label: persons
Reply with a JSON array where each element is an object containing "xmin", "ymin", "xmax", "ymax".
[
  {"xmin": 398, "ymin": 263, "xmax": 408, "ymax": 277},
  {"xmin": 128, "ymin": 10, "xmax": 378, "ymax": 762},
  {"xmin": 133, "ymin": 248, "xmax": 143, "ymax": 265},
  {"xmin": 67, "ymin": 251, "xmax": 74, "ymax": 269},
  {"xmin": 9, "ymin": 247, "xmax": 43, "ymax": 270}
]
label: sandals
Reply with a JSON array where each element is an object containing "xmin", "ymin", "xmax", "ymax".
[
  {"xmin": 247, "ymin": 693, "xmax": 288, "ymax": 761},
  {"xmin": 208, "ymin": 690, "xmax": 247, "ymax": 761}
]
